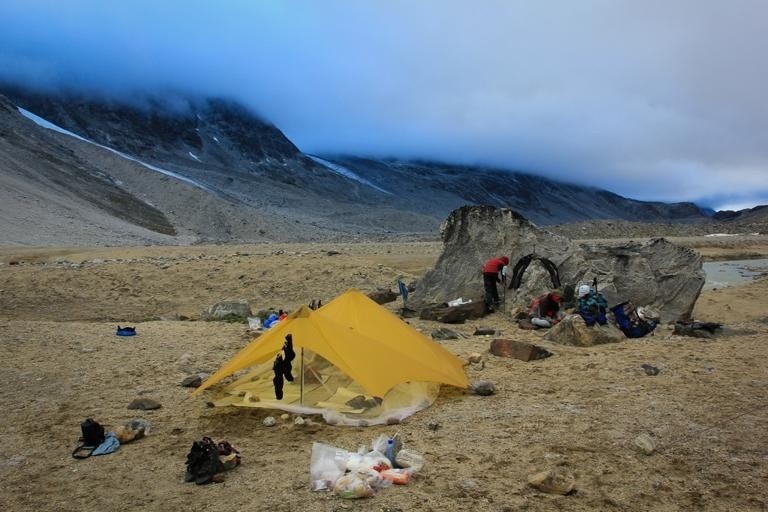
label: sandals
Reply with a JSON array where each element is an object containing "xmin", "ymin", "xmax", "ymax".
[{"xmin": 184, "ymin": 437, "xmax": 241, "ymax": 485}]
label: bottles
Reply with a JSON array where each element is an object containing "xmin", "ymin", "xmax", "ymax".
[{"xmin": 387, "ymin": 440, "xmax": 395, "ymax": 467}]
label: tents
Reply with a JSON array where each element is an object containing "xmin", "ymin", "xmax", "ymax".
[{"xmin": 179, "ymin": 284, "xmax": 474, "ymax": 428}]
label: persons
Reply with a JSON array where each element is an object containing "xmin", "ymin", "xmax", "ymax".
[
  {"xmin": 528, "ymin": 288, "xmax": 566, "ymax": 327},
  {"xmin": 481, "ymin": 256, "xmax": 509, "ymax": 305},
  {"xmin": 577, "ymin": 284, "xmax": 609, "ymax": 327}
]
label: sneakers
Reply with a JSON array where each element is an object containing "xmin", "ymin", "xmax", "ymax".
[
  {"xmin": 494, "ymin": 297, "xmax": 504, "ymax": 304},
  {"xmin": 488, "ymin": 304, "xmax": 494, "ymax": 312}
]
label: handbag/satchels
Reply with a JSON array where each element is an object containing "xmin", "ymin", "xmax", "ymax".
[{"xmin": 81, "ymin": 419, "xmax": 103, "ymax": 445}]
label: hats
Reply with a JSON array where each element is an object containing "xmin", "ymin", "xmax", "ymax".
[
  {"xmin": 551, "ymin": 289, "xmax": 561, "ymax": 296},
  {"xmin": 579, "ymin": 286, "xmax": 590, "ymax": 297}
]
